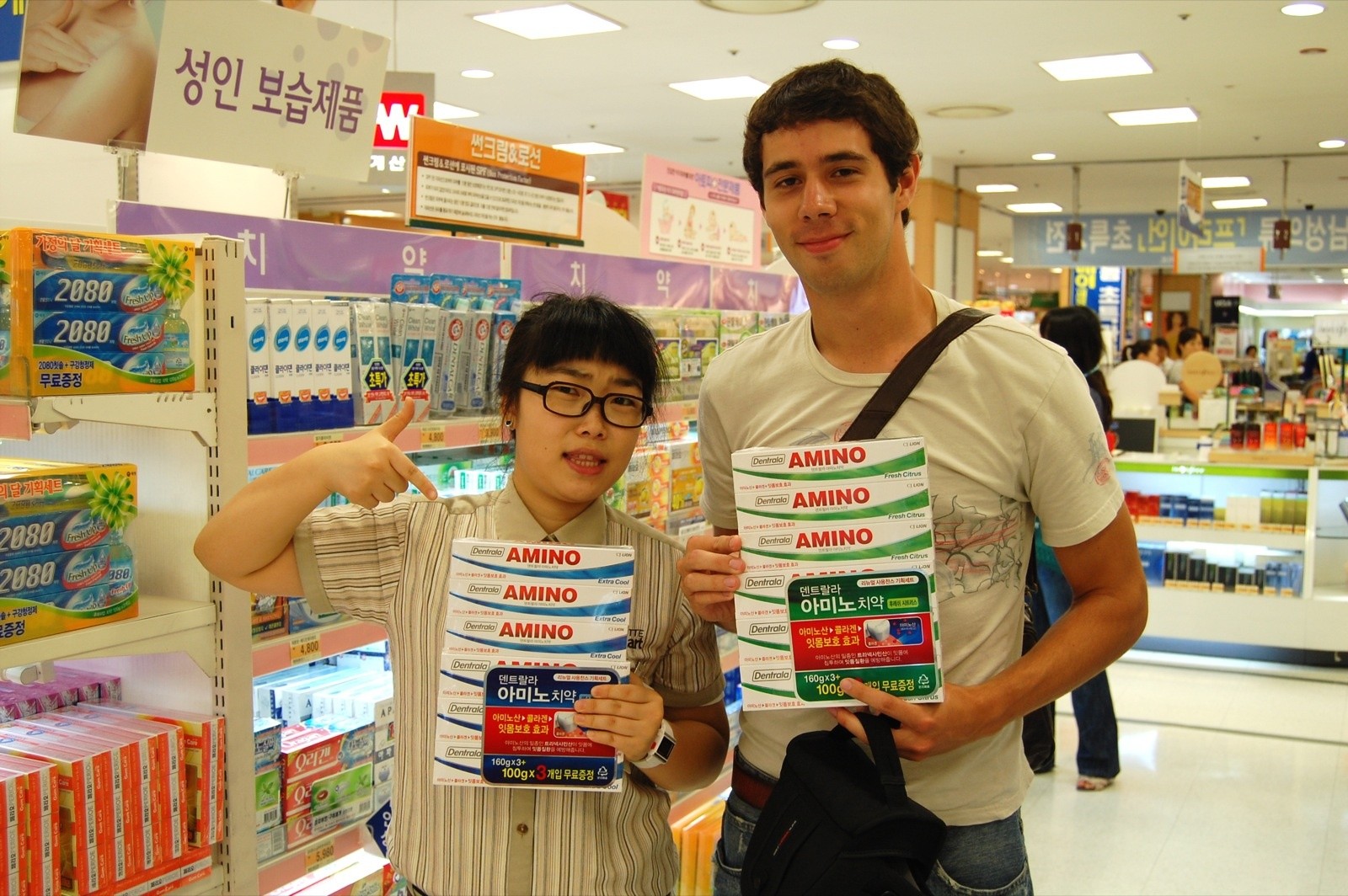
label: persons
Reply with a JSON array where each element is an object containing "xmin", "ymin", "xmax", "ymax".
[
  {"xmin": 676, "ymin": 57, "xmax": 1148, "ymax": 896},
  {"xmin": 17, "ymin": 0, "xmax": 159, "ymax": 151},
  {"xmin": 1022, "ymin": 306, "xmax": 1121, "ymax": 790},
  {"xmin": 193, "ymin": 291, "xmax": 730, "ymax": 896},
  {"xmin": 1106, "ymin": 328, "xmax": 1323, "ymax": 417}
]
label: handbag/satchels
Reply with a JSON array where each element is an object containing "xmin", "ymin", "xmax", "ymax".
[{"xmin": 741, "ymin": 711, "xmax": 947, "ymax": 896}]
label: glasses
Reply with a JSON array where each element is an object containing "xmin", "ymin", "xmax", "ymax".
[{"xmin": 517, "ymin": 381, "xmax": 654, "ymax": 428}]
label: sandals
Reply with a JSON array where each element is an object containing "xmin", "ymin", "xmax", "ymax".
[{"xmin": 1077, "ymin": 775, "xmax": 1112, "ymax": 792}]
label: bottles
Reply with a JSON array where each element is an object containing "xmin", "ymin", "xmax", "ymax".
[
  {"xmin": 109, "ymin": 531, "xmax": 134, "ymax": 606},
  {"xmin": 164, "ymin": 308, "xmax": 190, "ymax": 374},
  {"xmin": 0, "ymin": 285, "xmax": 10, "ymax": 365}
]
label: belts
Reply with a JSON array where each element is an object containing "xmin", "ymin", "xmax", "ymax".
[{"xmin": 731, "ymin": 750, "xmax": 776, "ymax": 812}]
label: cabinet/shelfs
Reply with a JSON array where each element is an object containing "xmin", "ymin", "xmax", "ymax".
[
  {"xmin": 0, "ymin": 232, "xmax": 741, "ymax": 896},
  {"xmin": 1112, "ymin": 450, "xmax": 1348, "ymax": 668}
]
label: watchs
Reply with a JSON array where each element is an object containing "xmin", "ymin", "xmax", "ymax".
[{"xmin": 631, "ymin": 718, "xmax": 675, "ymax": 768}]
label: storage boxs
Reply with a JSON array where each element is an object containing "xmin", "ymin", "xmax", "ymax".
[
  {"xmin": 0, "ymin": 228, "xmax": 779, "ymax": 896},
  {"xmin": 730, "ymin": 433, "xmax": 945, "ymax": 713}
]
